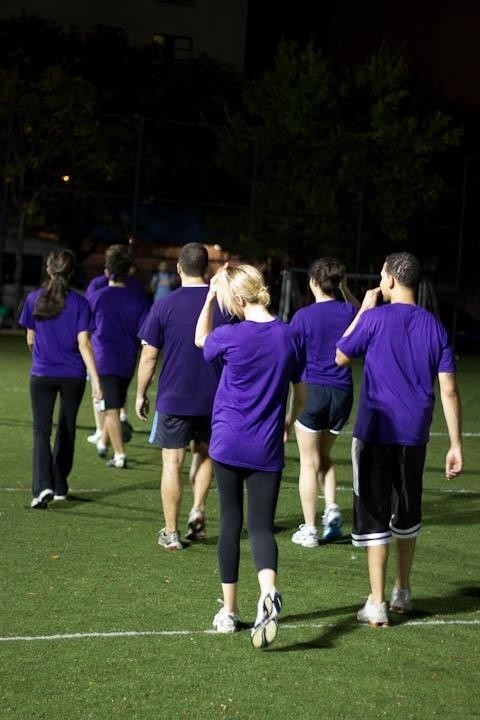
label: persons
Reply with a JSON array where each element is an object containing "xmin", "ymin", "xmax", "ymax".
[
  {"xmin": 291, "ymin": 257, "xmax": 360, "ymax": 547},
  {"xmin": 16, "ymin": 241, "xmax": 291, "ymax": 547},
  {"xmin": 194, "ymin": 263, "xmax": 303, "ymax": 647},
  {"xmin": 336, "ymin": 252, "xmax": 463, "ymax": 625}
]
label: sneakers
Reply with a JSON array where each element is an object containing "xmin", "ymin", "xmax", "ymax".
[
  {"xmin": 157, "ymin": 529, "xmax": 183, "ymax": 550},
  {"xmin": 356, "ymin": 599, "xmax": 388, "ymax": 628},
  {"xmin": 292, "ymin": 523, "xmax": 319, "ymax": 549},
  {"xmin": 212, "ymin": 605, "xmax": 239, "ymax": 635},
  {"xmin": 186, "ymin": 509, "xmax": 207, "ymax": 543},
  {"xmin": 390, "ymin": 588, "xmax": 414, "ymax": 615},
  {"xmin": 52, "ymin": 490, "xmax": 67, "ymax": 503},
  {"xmin": 320, "ymin": 505, "xmax": 345, "ymax": 543},
  {"xmin": 87, "ymin": 420, "xmax": 132, "ymax": 469},
  {"xmin": 252, "ymin": 590, "xmax": 283, "ymax": 649},
  {"xmin": 30, "ymin": 488, "xmax": 54, "ymax": 510}
]
